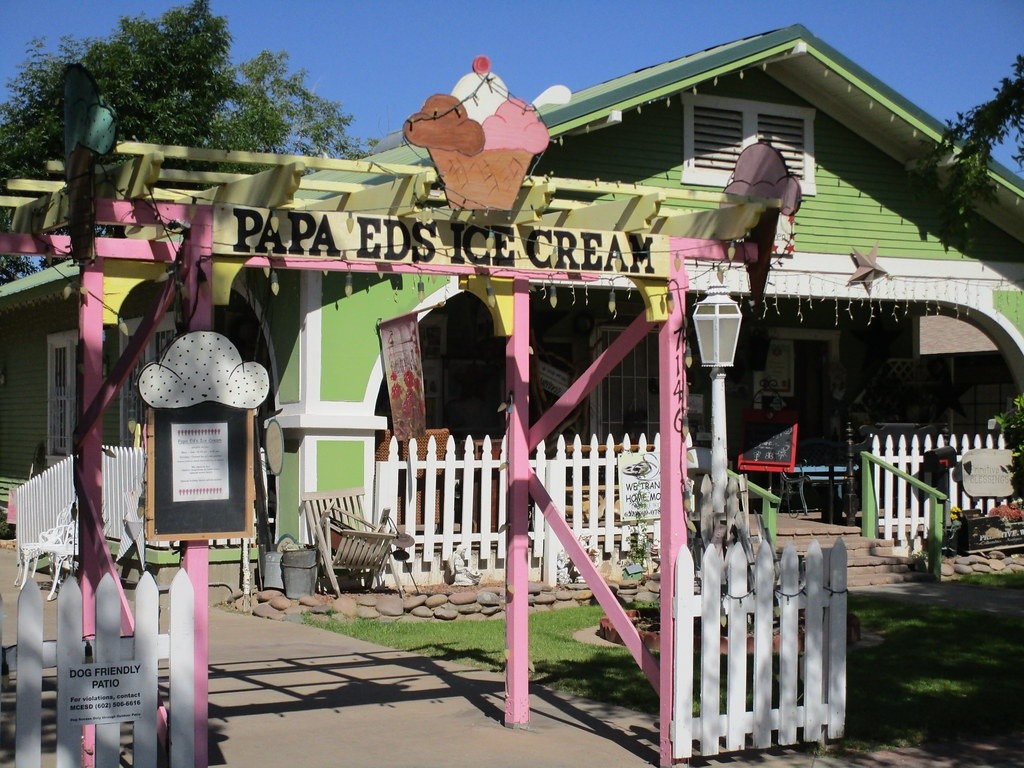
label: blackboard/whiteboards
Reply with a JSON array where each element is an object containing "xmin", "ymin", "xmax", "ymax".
[{"xmin": 737, "ymin": 409, "xmax": 799, "ymax": 471}]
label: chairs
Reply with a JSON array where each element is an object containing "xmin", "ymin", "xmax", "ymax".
[
  {"xmin": 304, "ymin": 486, "xmax": 408, "ymax": 602},
  {"xmin": 15, "ymin": 500, "xmax": 113, "ymax": 602}
]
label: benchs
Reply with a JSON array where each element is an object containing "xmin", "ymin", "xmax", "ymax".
[{"xmin": 776, "ymin": 437, "xmax": 858, "ymax": 525}]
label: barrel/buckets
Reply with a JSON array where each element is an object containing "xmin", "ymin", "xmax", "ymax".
[
  {"xmin": 282, "ymin": 545, "xmax": 318, "ymax": 599},
  {"xmin": 263, "ymin": 553, "xmax": 284, "ymax": 590}
]
